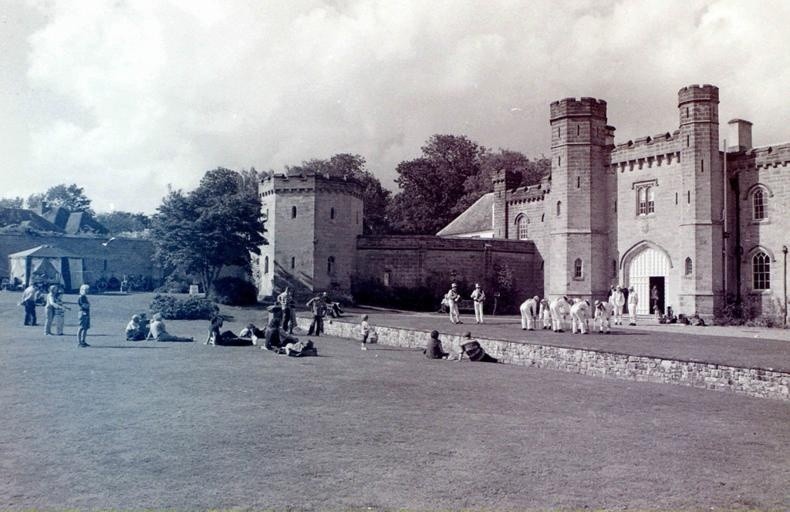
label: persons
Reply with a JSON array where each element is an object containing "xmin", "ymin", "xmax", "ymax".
[
  {"xmin": 422, "ymin": 329, "xmax": 449, "ymax": 359},
  {"xmin": 305, "ymin": 292, "xmax": 344, "ymax": 336},
  {"xmin": 125, "ymin": 312, "xmax": 194, "ymax": 342},
  {"xmin": 21, "ymin": 281, "xmax": 39, "ymax": 326},
  {"xmin": 457, "ymin": 330, "xmax": 502, "ymax": 363},
  {"xmin": 35, "ymin": 289, "xmax": 44, "ymax": 303},
  {"xmin": 440, "ymin": 280, "xmax": 659, "ymax": 334},
  {"xmin": 278, "ymin": 287, "xmax": 298, "ymax": 334},
  {"xmin": 44, "ymin": 285, "xmax": 71, "ymax": 336},
  {"xmin": 360, "ymin": 314, "xmax": 375, "ymax": 345},
  {"xmin": 77, "ymin": 284, "xmax": 91, "ymax": 347},
  {"xmin": 204, "ymin": 306, "xmax": 299, "ymax": 352}
]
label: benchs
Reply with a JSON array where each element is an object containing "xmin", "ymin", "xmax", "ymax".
[{"xmin": 457, "ymin": 300, "xmax": 474, "ymax": 311}]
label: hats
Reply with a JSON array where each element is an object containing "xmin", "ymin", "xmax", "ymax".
[
  {"xmin": 475, "ymin": 283, "xmax": 481, "ymax": 288},
  {"xmin": 451, "ymin": 282, "xmax": 457, "ymax": 288},
  {"xmin": 628, "ymin": 286, "xmax": 633, "ymax": 290},
  {"xmin": 534, "ymin": 295, "xmax": 602, "ymax": 307}
]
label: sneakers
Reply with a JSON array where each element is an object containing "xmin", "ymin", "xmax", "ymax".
[
  {"xmin": 523, "ymin": 326, "xmax": 610, "ymax": 334},
  {"xmin": 451, "ymin": 320, "xmax": 463, "ymax": 324},
  {"xmin": 361, "ymin": 347, "xmax": 368, "ymax": 350},
  {"xmin": 615, "ymin": 322, "xmax": 636, "ymax": 325},
  {"xmin": 78, "ymin": 342, "xmax": 90, "ymax": 347}
]
